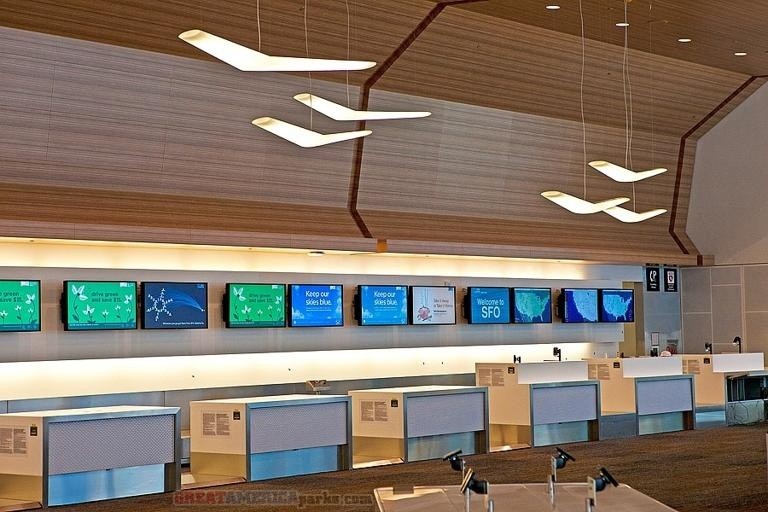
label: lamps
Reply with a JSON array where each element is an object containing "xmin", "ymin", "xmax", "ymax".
[
  {"xmin": 539, "ymin": 1, "xmax": 668, "ymax": 224},
  {"xmin": 177, "ymin": 0, "xmax": 431, "ymax": 149}
]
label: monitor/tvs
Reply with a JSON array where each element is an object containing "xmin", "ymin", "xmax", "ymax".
[
  {"xmin": 600, "ymin": 288, "xmax": 636, "ymax": 324},
  {"xmin": 511, "ymin": 287, "xmax": 552, "ymax": 323},
  {"xmin": 63, "ymin": 279, "xmax": 137, "ymax": 330},
  {"xmin": 141, "ymin": 280, "xmax": 209, "ymax": 329},
  {"xmin": 291, "ymin": 282, "xmax": 345, "ymax": 327},
  {"xmin": 463, "ymin": 286, "xmax": 509, "ymax": 324},
  {"xmin": 0, "ymin": 279, "xmax": 40, "ymax": 332},
  {"xmin": 221, "ymin": 282, "xmax": 287, "ymax": 326},
  {"xmin": 355, "ymin": 283, "xmax": 409, "ymax": 326},
  {"xmin": 410, "ymin": 285, "xmax": 456, "ymax": 325},
  {"xmin": 559, "ymin": 287, "xmax": 599, "ymax": 323}
]
